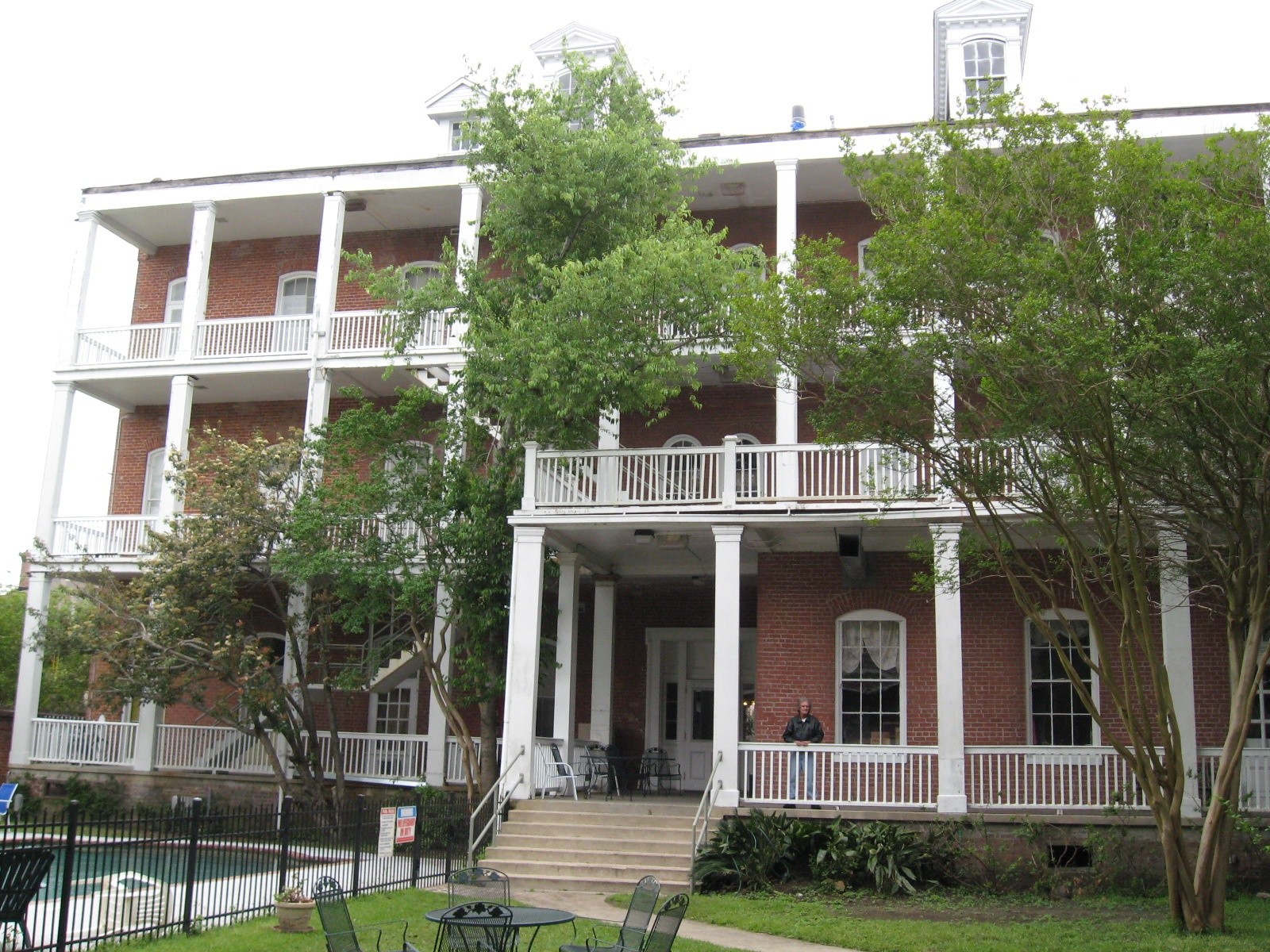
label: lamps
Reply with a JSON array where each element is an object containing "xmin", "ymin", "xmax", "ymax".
[
  {"xmin": 345, "ymin": 198, "xmax": 367, "ymax": 212},
  {"xmin": 720, "ymin": 181, "xmax": 745, "ymax": 195},
  {"xmin": 634, "ymin": 529, "xmax": 654, "ymax": 545},
  {"xmin": 653, "ymin": 532, "xmax": 689, "ymax": 550}
]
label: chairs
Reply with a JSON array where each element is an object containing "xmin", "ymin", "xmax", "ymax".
[
  {"xmin": 539, "ymin": 741, "xmax": 682, "ymax": 802},
  {"xmin": 0, "ymin": 849, "xmax": 56, "ymax": 948},
  {"xmin": 0, "ymin": 783, "xmax": 18, "ymax": 833},
  {"xmin": 311, "ymin": 867, "xmax": 690, "ymax": 952}
]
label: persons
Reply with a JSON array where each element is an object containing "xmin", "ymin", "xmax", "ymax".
[{"xmin": 783, "ymin": 696, "xmax": 824, "ymax": 809}]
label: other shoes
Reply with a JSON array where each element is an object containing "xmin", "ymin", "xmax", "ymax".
[
  {"xmin": 810, "ymin": 805, "xmax": 821, "ymax": 809},
  {"xmin": 783, "ymin": 804, "xmax": 796, "ymax": 809}
]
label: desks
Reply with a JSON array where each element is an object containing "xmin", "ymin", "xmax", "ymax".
[
  {"xmin": 426, "ymin": 905, "xmax": 577, "ymax": 952},
  {"xmin": 580, "ymin": 755, "xmax": 676, "ymax": 801}
]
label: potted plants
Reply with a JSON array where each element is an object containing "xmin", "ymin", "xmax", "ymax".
[{"xmin": 274, "ymin": 886, "xmax": 315, "ymax": 932}]
